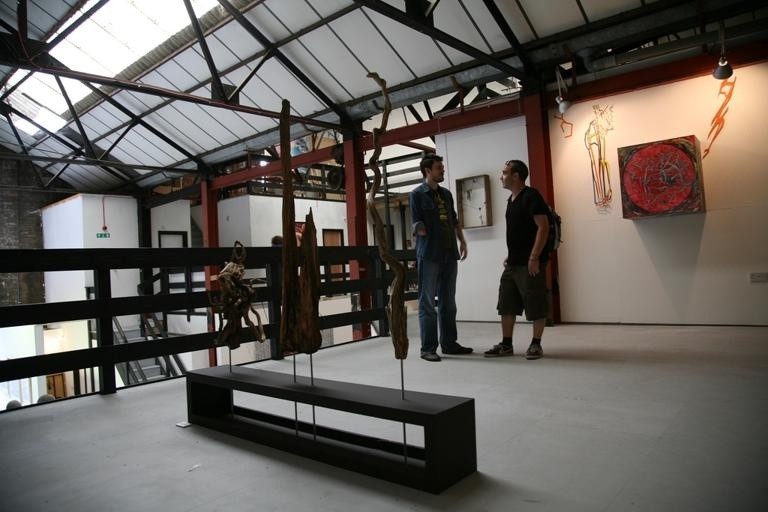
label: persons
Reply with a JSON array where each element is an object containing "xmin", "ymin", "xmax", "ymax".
[
  {"xmin": 483, "ymin": 159, "xmax": 550, "ymax": 360},
  {"xmin": 407, "ymin": 152, "xmax": 474, "ymax": 362}
]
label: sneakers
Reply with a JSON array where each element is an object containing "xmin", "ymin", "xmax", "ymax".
[
  {"xmin": 525, "ymin": 343, "xmax": 543, "ymax": 359},
  {"xmin": 483, "ymin": 343, "xmax": 514, "ymax": 357}
]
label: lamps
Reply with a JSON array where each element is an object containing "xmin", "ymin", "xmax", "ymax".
[
  {"xmin": 553, "ymin": 65, "xmax": 571, "ymax": 115},
  {"xmin": 698, "ymin": 44, "xmax": 733, "ymax": 79}
]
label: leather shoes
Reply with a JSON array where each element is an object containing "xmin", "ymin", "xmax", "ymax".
[
  {"xmin": 442, "ymin": 344, "xmax": 473, "ymax": 354},
  {"xmin": 420, "ymin": 352, "xmax": 441, "ymax": 361}
]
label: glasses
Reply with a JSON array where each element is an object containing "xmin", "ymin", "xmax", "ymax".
[{"xmin": 504, "ymin": 160, "xmax": 510, "ymax": 168}]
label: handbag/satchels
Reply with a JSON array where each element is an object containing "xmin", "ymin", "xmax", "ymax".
[{"xmin": 545, "ymin": 204, "xmax": 563, "ymax": 252}]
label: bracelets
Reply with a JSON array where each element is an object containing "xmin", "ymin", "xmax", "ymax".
[{"xmin": 529, "ymin": 254, "xmax": 539, "ymax": 260}]
